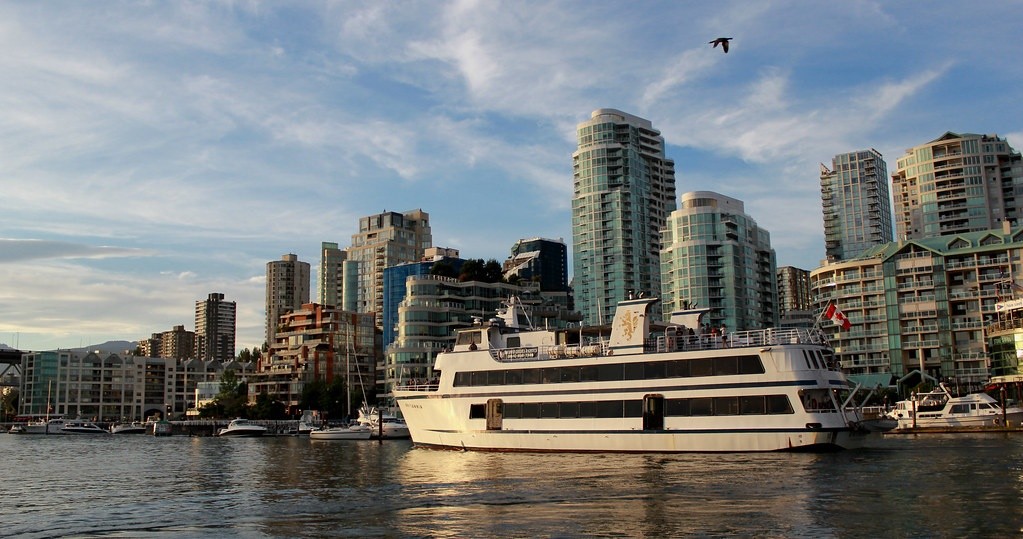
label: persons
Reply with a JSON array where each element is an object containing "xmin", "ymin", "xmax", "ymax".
[
  {"xmin": 675, "ymin": 321, "xmax": 730, "ymax": 351},
  {"xmin": 405, "ymin": 377, "xmax": 439, "ymax": 391},
  {"xmin": 923, "ymin": 399, "xmax": 945, "ymax": 406}
]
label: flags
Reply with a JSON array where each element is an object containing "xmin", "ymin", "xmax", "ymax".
[{"xmin": 824, "ymin": 303, "xmax": 851, "ymax": 331}]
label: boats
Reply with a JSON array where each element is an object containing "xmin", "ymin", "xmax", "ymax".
[
  {"xmin": 880, "ymin": 390, "xmax": 1023, "ymax": 432},
  {"xmin": 218, "ymin": 418, "xmax": 267, "ymax": 437},
  {"xmin": 389, "ymin": 290, "xmax": 865, "ymax": 454},
  {"xmin": 61, "ymin": 418, "xmax": 109, "ymax": 435},
  {"xmin": 359, "ymin": 403, "xmax": 410, "ymax": 438},
  {"xmin": 153, "ymin": 421, "xmax": 172, "ymax": 435},
  {"xmin": 310, "ymin": 423, "xmax": 372, "ymax": 440},
  {"xmin": 111, "ymin": 421, "xmax": 146, "ymax": 435}
]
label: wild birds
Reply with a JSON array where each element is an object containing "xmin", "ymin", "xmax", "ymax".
[{"xmin": 709, "ymin": 38, "xmax": 733, "ymax": 53}]
